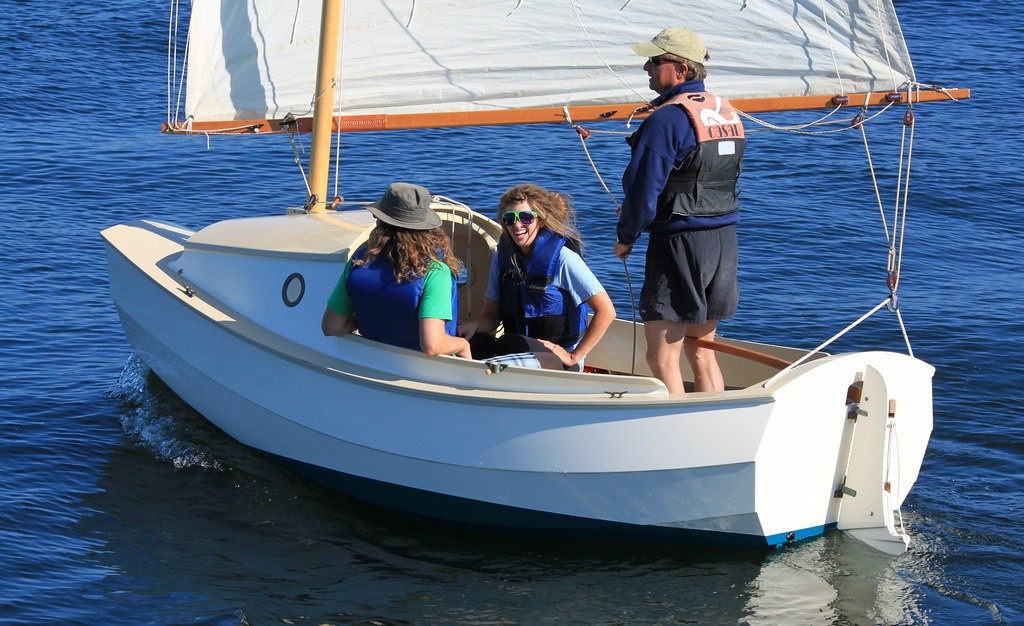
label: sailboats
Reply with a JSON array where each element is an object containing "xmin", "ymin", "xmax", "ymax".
[{"xmin": 98, "ymin": 0, "xmax": 973, "ymax": 557}]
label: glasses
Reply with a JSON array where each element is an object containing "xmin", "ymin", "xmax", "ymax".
[
  {"xmin": 502, "ymin": 210, "xmax": 539, "ymax": 226},
  {"xmin": 648, "ymin": 56, "xmax": 675, "ymax": 65}
]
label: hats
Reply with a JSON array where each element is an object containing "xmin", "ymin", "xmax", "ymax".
[
  {"xmin": 631, "ymin": 26, "xmax": 711, "ymax": 66},
  {"xmin": 368, "ymin": 182, "xmax": 443, "ymax": 229}
]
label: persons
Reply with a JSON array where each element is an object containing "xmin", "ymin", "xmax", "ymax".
[
  {"xmin": 466, "ymin": 184, "xmax": 618, "ymax": 373},
  {"xmin": 322, "ymin": 182, "xmax": 472, "ymax": 363},
  {"xmin": 613, "ymin": 25, "xmax": 746, "ymax": 398}
]
